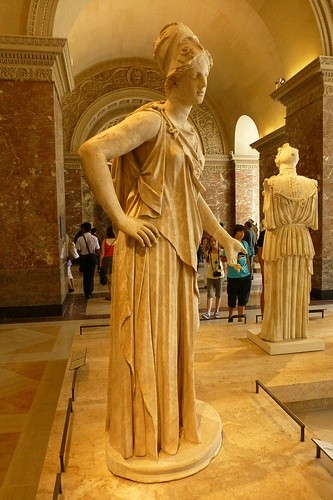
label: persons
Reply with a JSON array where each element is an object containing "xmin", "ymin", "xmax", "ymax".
[
  {"xmin": 197, "ymin": 219, "xmax": 258, "ymax": 280},
  {"xmin": 260, "ymin": 142, "xmax": 318, "ymax": 342},
  {"xmin": 66, "ymin": 222, "xmax": 115, "ymax": 300},
  {"xmin": 221, "ymin": 225, "xmax": 251, "ymax": 322},
  {"xmin": 257, "ymin": 230, "xmax": 266, "ymax": 320},
  {"xmin": 202, "ymin": 236, "xmax": 225, "ymax": 320},
  {"xmin": 78, "ymin": 21, "xmax": 248, "ymax": 458}
]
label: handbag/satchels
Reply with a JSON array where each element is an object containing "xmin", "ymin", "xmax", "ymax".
[
  {"xmin": 87, "ymin": 254, "xmax": 97, "ymax": 265},
  {"xmin": 100, "ymin": 268, "xmax": 107, "ymax": 285},
  {"xmin": 67, "ymin": 241, "xmax": 79, "ymax": 260}
]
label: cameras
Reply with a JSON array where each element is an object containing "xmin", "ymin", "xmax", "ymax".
[{"xmin": 213, "ymin": 271, "xmax": 221, "ymax": 277}]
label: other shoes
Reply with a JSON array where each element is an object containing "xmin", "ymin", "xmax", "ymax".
[
  {"xmin": 214, "ymin": 312, "xmax": 219, "ymax": 318},
  {"xmin": 69, "ymin": 288, "xmax": 74, "ymax": 292},
  {"xmin": 87, "ymin": 295, "xmax": 91, "ymax": 298},
  {"xmin": 105, "ymin": 296, "xmax": 110, "ymax": 299},
  {"xmin": 202, "ymin": 313, "xmax": 210, "ymax": 320}
]
